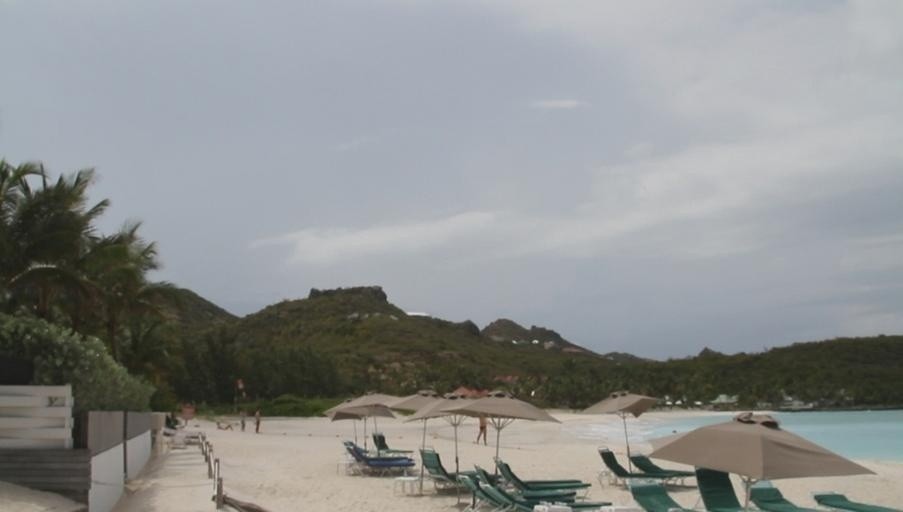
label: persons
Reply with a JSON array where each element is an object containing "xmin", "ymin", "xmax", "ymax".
[
  {"xmin": 254, "ymin": 407, "xmax": 261, "ymax": 434},
  {"xmin": 238, "ymin": 408, "xmax": 249, "ymax": 431},
  {"xmin": 475, "ymin": 413, "xmax": 489, "ymax": 447}
]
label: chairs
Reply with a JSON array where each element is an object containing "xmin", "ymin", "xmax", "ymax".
[
  {"xmin": 597, "ymin": 446, "xmax": 646, "ymax": 490},
  {"xmin": 164, "ymin": 427, "xmax": 208, "ymax": 450},
  {"xmin": 626, "ymin": 449, "xmax": 696, "ymax": 491},
  {"xmin": 343, "ymin": 433, "xmax": 415, "ymax": 478},
  {"xmin": 810, "ymin": 488, "xmax": 903, "ymax": 511},
  {"xmin": 459, "ymin": 455, "xmax": 613, "ymax": 511},
  {"xmin": 694, "ymin": 464, "xmax": 750, "ymax": 512},
  {"xmin": 628, "ymin": 475, "xmax": 698, "ymax": 512},
  {"xmin": 740, "ymin": 478, "xmax": 825, "ymax": 512},
  {"xmin": 418, "ymin": 447, "xmax": 475, "ymax": 496}
]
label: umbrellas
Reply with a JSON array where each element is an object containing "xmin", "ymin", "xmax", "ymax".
[
  {"xmin": 324, "ymin": 397, "xmax": 359, "ymax": 447},
  {"xmin": 405, "ymin": 399, "xmax": 476, "ymax": 506},
  {"xmin": 577, "ymin": 386, "xmax": 666, "ymax": 474},
  {"xmin": 648, "ymin": 408, "xmax": 879, "ymax": 512},
  {"xmin": 343, "ymin": 393, "xmax": 399, "ymax": 456},
  {"xmin": 330, "ymin": 402, "xmax": 392, "ymax": 450},
  {"xmin": 440, "ymin": 398, "xmax": 564, "ymax": 482},
  {"xmin": 387, "ymin": 393, "xmax": 444, "ymax": 491}
]
